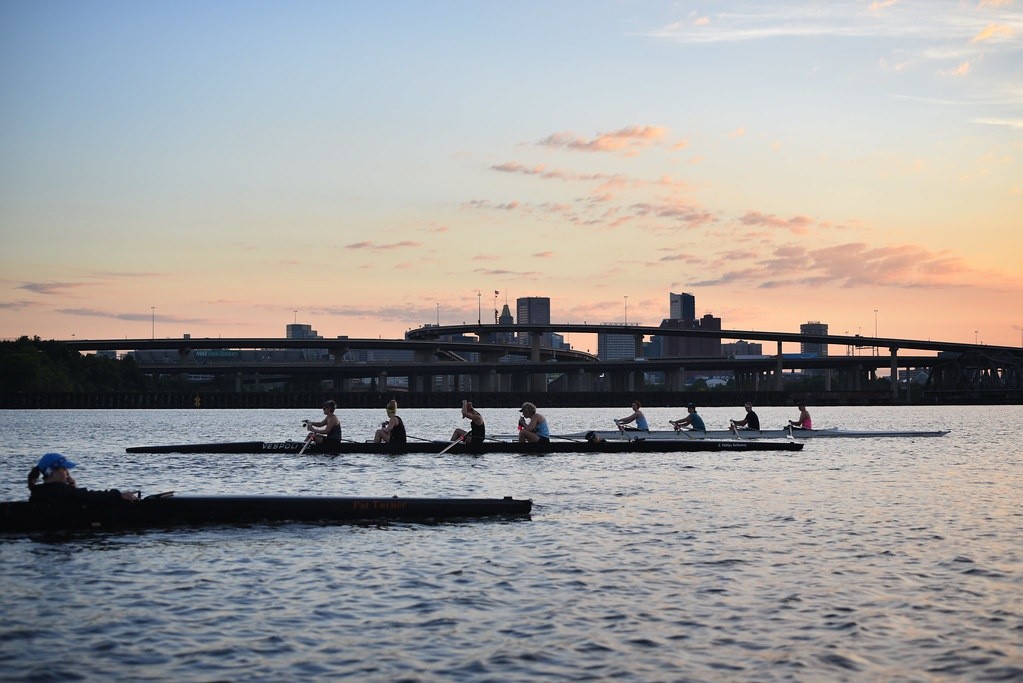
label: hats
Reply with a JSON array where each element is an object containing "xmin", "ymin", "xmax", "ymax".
[
  {"xmin": 387, "ymin": 400, "xmax": 396, "ymax": 412},
  {"xmin": 38, "ymin": 453, "xmax": 77, "ymax": 475},
  {"xmin": 519, "ymin": 403, "xmax": 536, "ymax": 411},
  {"xmin": 686, "ymin": 403, "xmax": 695, "ymax": 407}
]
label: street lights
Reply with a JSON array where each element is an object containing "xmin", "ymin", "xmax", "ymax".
[
  {"xmin": 974, "ymin": 330, "xmax": 979, "ymax": 345},
  {"xmin": 72, "ymin": 334, "xmax": 75, "ymax": 340},
  {"xmin": 845, "ymin": 331, "xmax": 848, "ymax": 354},
  {"xmin": 151, "ymin": 306, "xmax": 156, "ymax": 339},
  {"xmin": 624, "ymin": 295, "xmax": 628, "ymax": 325},
  {"xmin": 874, "ymin": 309, "xmax": 879, "ymax": 338},
  {"xmin": 294, "ymin": 310, "xmax": 298, "ymax": 324}
]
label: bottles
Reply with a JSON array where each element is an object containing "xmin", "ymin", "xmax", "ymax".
[
  {"xmin": 518, "ymin": 417, "xmax": 523, "ymax": 430},
  {"xmin": 382, "ymin": 424, "xmax": 386, "ymax": 429},
  {"xmin": 308, "ymin": 420, "xmax": 312, "ymax": 430}
]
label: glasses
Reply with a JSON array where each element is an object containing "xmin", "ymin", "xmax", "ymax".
[{"xmin": 323, "ymin": 407, "xmax": 327, "ymax": 410}]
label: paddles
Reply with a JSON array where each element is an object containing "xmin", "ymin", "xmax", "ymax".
[
  {"xmin": 298, "ymin": 432, "xmax": 317, "ymax": 455},
  {"xmin": 669, "ymin": 420, "xmax": 693, "ymax": 439},
  {"xmin": 731, "ymin": 419, "xmax": 746, "ymax": 440},
  {"xmin": 614, "ymin": 419, "xmax": 633, "ymax": 440},
  {"xmin": 406, "ymin": 435, "xmax": 431, "ymax": 441},
  {"xmin": 438, "ymin": 430, "xmax": 472, "ymax": 455},
  {"xmin": 786, "ymin": 420, "xmax": 794, "ymax": 440},
  {"xmin": 484, "ymin": 437, "xmax": 500, "ymax": 442},
  {"xmin": 551, "ymin": 435, "xmax": 579, "ymax": 442},
  {"xmin": 302, "ymin": 419, "xmax": 359, "ymax": 443}
]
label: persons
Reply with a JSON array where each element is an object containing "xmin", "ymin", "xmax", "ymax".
[
  {"xmin": 519, "ymin": 402, "xmax": 550, "ymax": 443},
  {"xmin": 29, "ymin": 453, "xmax": 139, "ymax": 503},
  {"xmin": 304, "ymin": 400, "xmax": 342, "ymax": 444},
  {"xmin": 450, "ymin": 401, "xmax": 485, "ymax": 443},
  {"xmin": 784, "ymin": 402, "xmax": 812, "ymax": 429},
  {"xmin": 669, "ymin": 403, "xmax": 706, "ymax": 432},
  {"xmin": 614, "ymin": 401, "xmax": 648, "ymax": 432},
  {"xmin": 730, "ymin": 402, "xmax": 761, "ymax": 430},
  {"xmin": 374, "ymin": 400, "xmax": 406, "ymax": 443}
]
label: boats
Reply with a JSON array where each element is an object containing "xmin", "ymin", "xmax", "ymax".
[
  {"xmin": 0, "ymin": 490, "xmax": 535, "ymax": 534},
  {"xmin": 125, "ymin": 440, "xmax": 808, "ymax": 455},
  {"xmin": 489, "ymin": 426, "xmax": 956, "ymax": 439}
]
